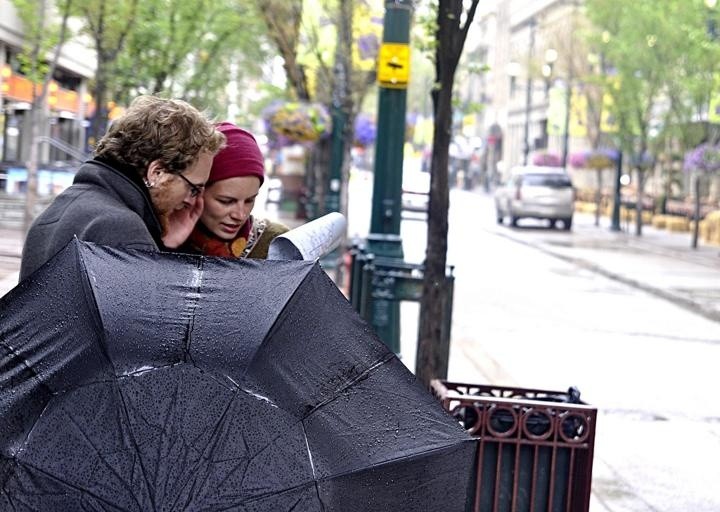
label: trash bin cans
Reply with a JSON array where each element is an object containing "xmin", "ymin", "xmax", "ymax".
[{"xmin": 427, "ymin": 378, "xmax": 598, "ymax": 512}]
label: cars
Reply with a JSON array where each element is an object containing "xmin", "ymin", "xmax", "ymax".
[
  {"xmin": 494, "ymin": 165, "xmax": 574, "ymax": 230},
  {"xmin": 401, "ymin": 173, "xmax": 431, "ymax": 212}
]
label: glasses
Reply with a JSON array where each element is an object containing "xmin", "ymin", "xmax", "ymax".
[{"xmin": 166, "ymin": 169, "xmax": 201, "ymax": 198}]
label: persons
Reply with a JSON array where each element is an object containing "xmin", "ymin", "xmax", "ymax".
[
  {"xmin": 177, "ymin": 119, "xmax": 295, "ymax": 261},
  {"xmin": 18, "ymin": 93, "xmax": 226, "ymax": 284}
]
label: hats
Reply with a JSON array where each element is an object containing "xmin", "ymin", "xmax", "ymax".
[{"xmin": 204, "ymin": 122, "xmax": 264, "ymax": 188}]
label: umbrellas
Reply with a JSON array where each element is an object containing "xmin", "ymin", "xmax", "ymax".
[{"xmin": 0, "ymin": 233, "xmax": 482, "ymax": 512}]
